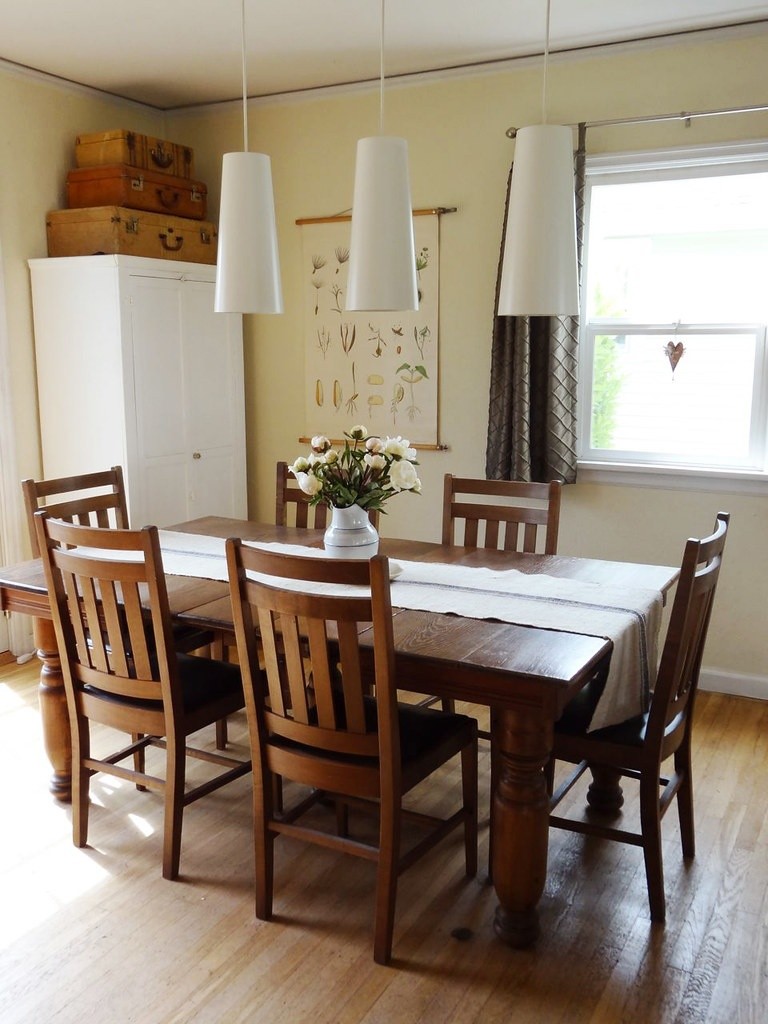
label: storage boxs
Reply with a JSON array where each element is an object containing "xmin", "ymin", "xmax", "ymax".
[{"xmin": 45, "ymin": 128, "xmax": 217, "ymax": 265}]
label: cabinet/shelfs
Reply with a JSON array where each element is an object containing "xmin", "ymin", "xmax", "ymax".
[{"xmin": 26, "ymin": 254, "xmax": 248, "ymax": 529}]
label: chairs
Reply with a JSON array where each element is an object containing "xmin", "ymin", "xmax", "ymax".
[{"xmin": 22, "ymin": 461, "xmax": 731, "ymax": 965}]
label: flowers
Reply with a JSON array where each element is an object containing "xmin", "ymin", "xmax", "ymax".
[{"xmin": 287, "ymin": 424, "xmax": 422, "ymax": 515}]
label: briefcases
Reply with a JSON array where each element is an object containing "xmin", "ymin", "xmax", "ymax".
[
  {"xmin": 75, "ymin": 129, "xmax": 195, "ymax": 178},
  {"xmin": 45, "ymin": 204, "xmax": 217, "ymax": 264},
  {"xmin": 68, "ymin": 163, "xmax": 207, "ymax": 219}
]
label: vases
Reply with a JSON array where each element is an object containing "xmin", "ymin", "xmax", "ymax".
[{"xmin": 323, "ymin": 504, "xmax": 379, "ymax": 559}]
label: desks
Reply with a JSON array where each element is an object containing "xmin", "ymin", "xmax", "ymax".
[{"xmin": 0, "ymin": 516, "xmax": 682, "ymax": 948}]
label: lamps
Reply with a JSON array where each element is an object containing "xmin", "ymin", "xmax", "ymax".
[
  {"xmin": 214, "ymin": 0, "xmax": 284, "ymax": 314},
  {"xmin": 346, "ymin": 0, "xmax": 419, "ymax": 311},
  {"xmin": 498, "ymin": 0, "xmax": 581, "ymax": 315}
]
